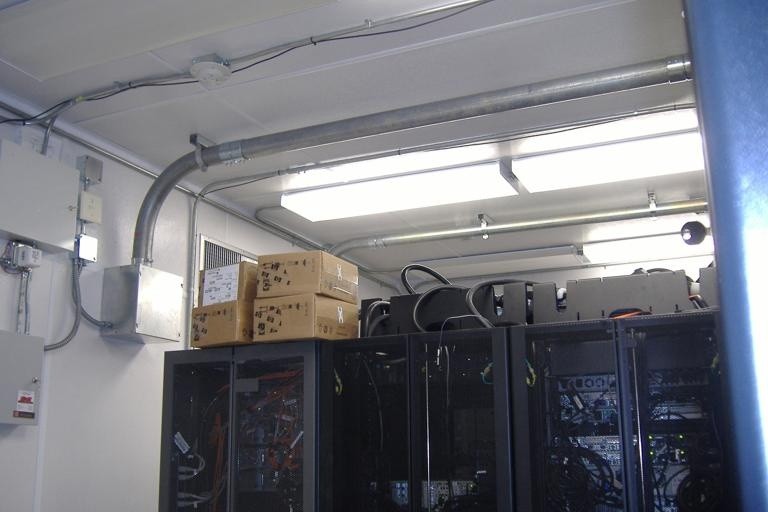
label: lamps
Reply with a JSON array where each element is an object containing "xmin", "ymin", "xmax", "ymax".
[{"xmin": 279, "ymin": 108, "xmax": 715, "ymax": 283}]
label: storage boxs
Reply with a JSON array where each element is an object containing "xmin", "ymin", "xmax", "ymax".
[{"xmin": 189, "ymin": 250, "xmax": 359, "ymax": 349}]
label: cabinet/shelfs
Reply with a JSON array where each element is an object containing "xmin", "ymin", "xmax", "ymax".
[{"xmin": 158, "ymin": 308, "xmax": 738, "ymax": 512}]
